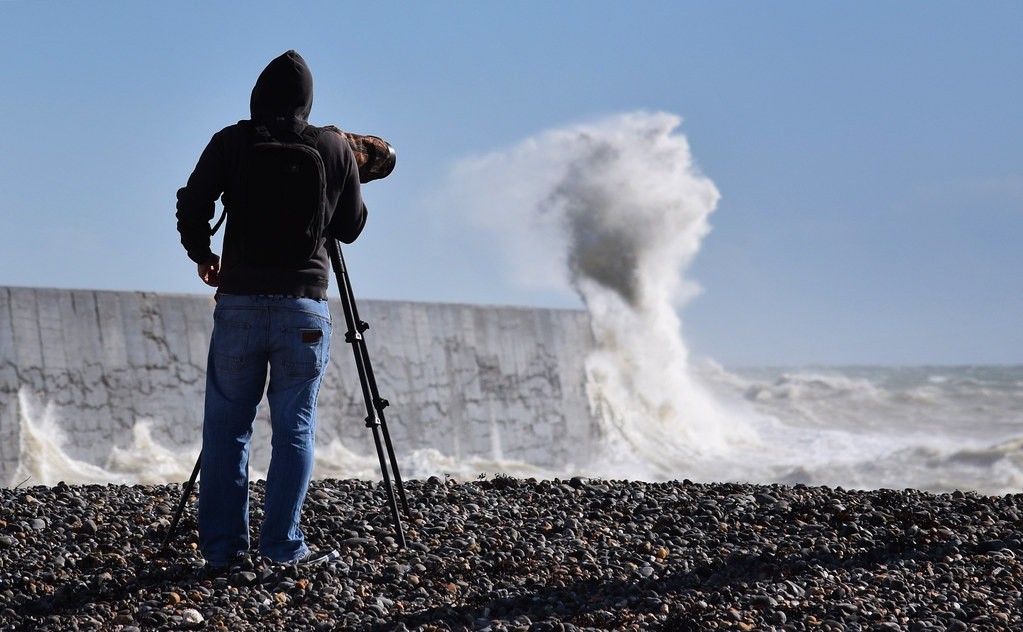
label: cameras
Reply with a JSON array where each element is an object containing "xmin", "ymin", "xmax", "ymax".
[{"xmin": 318, "ymin": 125, "xmax": 396, "ymax": 183}]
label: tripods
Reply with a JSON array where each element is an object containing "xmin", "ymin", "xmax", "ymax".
[{"xmin": 158, "ymin": 233, "xmax": 416, "ymax": 556}]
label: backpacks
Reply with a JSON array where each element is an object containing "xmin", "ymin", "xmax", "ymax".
[{"xmin": 226, "ymin": 118, "xmax": 330, "ymax": 268}]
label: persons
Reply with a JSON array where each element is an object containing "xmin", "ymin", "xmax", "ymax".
[{"xmin": 173, "ymin": 49, "xmax": 370, "ymax": 576}]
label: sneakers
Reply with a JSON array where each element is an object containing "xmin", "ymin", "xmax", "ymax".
[{"xmin": 286, "ymin": 548, "xmax": 339, "ymax": 567}]
